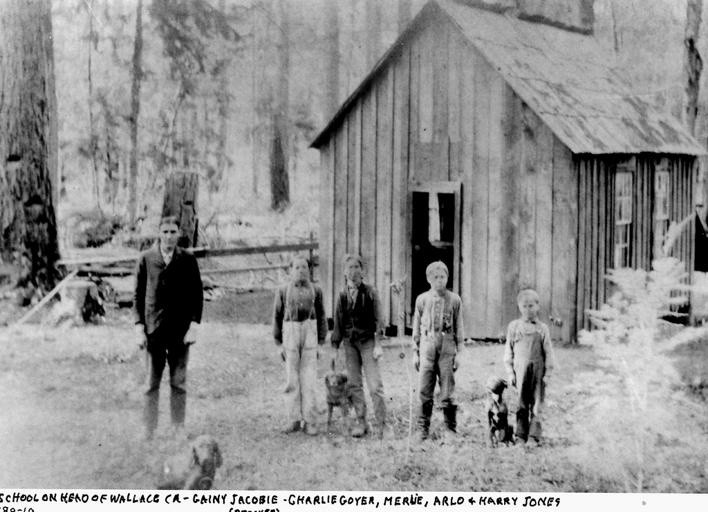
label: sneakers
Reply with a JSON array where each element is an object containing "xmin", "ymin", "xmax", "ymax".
[{"xmin": 271, "ymin": 419, "xmax": 543, "ymax": 451}]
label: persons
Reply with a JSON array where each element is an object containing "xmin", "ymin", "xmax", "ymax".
[
  {"xmin": 502, "ymin": 286, "xmax": 557, "ymax": 451},
  {"xmin": 131, "ymin": 214, "xmax": 205, "ymax": 444},
  {"xmin": 409, "ymin": 257, "xmax": 468, "ymax": 443},
  {"xmin": 270, "ymin": 254, "xmax": 330, "ymax": 438},
  {"xmin": 329, "ymin": 252, "xmax": 390, "ymax": 440}
]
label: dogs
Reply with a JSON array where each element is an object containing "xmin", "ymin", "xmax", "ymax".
[
  {"xmin": 324, "ymin": 371, "xmax": 351, "ymax": 434},
  {"xmin": 151, "ymin": 434, "xmax": 225, "ymax": 490},
  {"xmin": 486, "ymin": 378, "xmax": 516, "ymax": 449}
]
